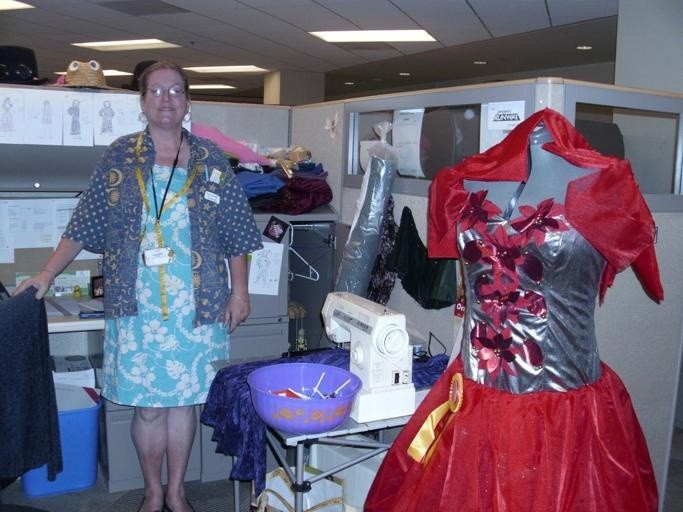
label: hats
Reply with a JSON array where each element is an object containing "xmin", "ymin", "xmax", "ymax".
[
  {"xmin": 0, "ymin": 46, "xmax": 48, "ymax": 85},
  {"xmin": 48, "ymin": 60, "xmax": 119, "ymax": 91}
]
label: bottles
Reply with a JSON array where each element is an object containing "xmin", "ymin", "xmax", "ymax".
[{"xmin": 73, "ymin": 285, "xmax": 80, "ymax": 299}]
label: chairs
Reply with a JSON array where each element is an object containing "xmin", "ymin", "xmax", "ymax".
[{"xmin": 0, "ymin": 283, "xmax": 69, "ymax": 511}]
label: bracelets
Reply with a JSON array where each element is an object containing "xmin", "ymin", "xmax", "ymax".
[
  {"xmin": 40, "ymin": 269, "xmax": 57, "ymax": 280},
  {"xmin": 232, "ymin": 292, "xmax": 249, "ymax": 304}
]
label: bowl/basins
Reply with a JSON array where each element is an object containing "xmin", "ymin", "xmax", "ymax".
[{"xmin": 246, "ymin": 363, "xmax": 363, "ymax": 435}]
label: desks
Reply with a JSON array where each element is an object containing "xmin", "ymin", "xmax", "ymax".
[{"xmin": 216, "ymin": 348, "xmax": 433, "ymax": 512}]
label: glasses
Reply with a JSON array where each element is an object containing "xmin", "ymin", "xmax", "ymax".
[{"xmin": 144, "ymin": 86, "xmax": 186, "ymax": 98}]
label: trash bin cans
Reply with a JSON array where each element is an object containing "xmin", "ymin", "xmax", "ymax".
[{"xmin": 21, "ymin": 397, "xmax": 104, "ymax": 496}]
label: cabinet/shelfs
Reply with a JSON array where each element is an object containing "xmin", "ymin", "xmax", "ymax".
[{"xmin": 39, "ymin": 197, "xmax": 340, "ymax": 496}]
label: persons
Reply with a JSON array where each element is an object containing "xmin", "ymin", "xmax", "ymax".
[
  {"xmin": 364, "ymin": 108, "xmax": 665, "ymax": 512},
  {"xmin": 10, "ymin": 59, "xmax": 264, "ymax": 512}
]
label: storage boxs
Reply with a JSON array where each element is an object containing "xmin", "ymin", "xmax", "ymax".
[
  {"xmin": 247, "ymin": 460, "xmax": 344, "ymax": 512},
  {"xmin": 16, "ymin": 382, "xmax": 103, "ymax": 501},
  {"xmin": 306, "ymin": 429, "xmax": 393, "ymax": 509}
]
